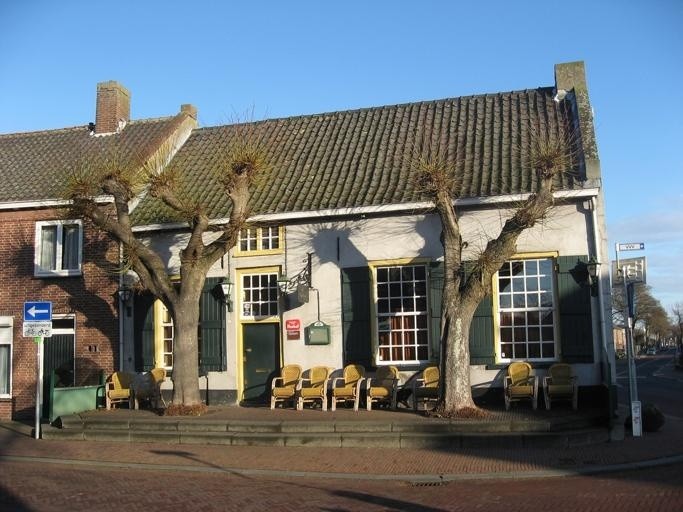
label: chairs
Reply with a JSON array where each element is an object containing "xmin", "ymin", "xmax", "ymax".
[
  {"xmin": 106, "ymin": 371, "xmax": 136, "ymax": 411},
  {"xmin": 271, "ymin": 364, "xmax": 439, "ymax": 412},
  {"xmin": 503, "ymin": 362, "xmax": 539, "ymax": 411},
  {"xmin": 135, "ymin": 368, "xmax": 167, "ymax": 410},
  {"xmin": 542, "ymin": 363, "xmax": 579, "ymax": 410}
]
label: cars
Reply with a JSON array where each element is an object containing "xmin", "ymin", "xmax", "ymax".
[
  {"xmin": 646, "ymin": 347, "xmax": 656, "ymax": 355},
  {"xmin": 660, "ymin": 347, "xmax": 665, "ymax": 352}
]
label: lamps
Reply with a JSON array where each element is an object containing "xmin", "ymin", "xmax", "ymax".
[
  {"xmin": 275, "ymin": 274, "xmax": 290, "ymax": 310},
  {"xmin": 118, "ymin": 284, "xmax": 132, "ymax": 317},
  {"xmin": 586, "ymin": 253, "xmax": 601, "ymax": 296},
  {"xmin": 218, "ymin": 277, "xmax": 233, "ymax": 312}
]
